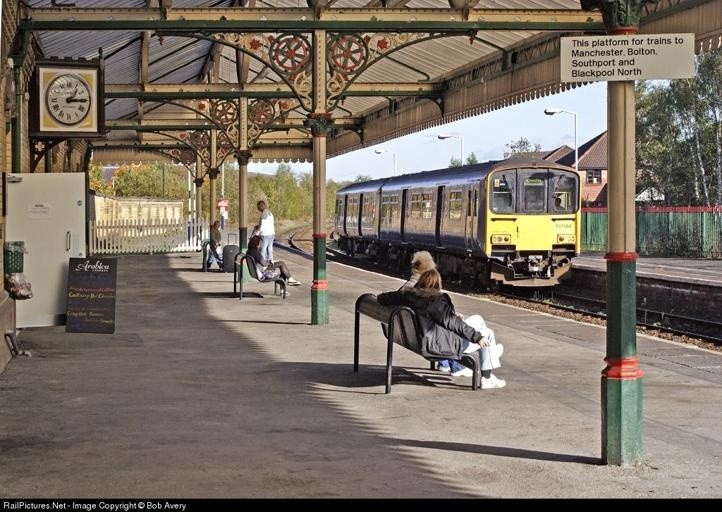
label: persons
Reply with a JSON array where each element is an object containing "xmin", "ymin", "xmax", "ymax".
[
  {"xmin": 207, "ymin": 221, "xmax": 223, "ymax": 271},
  {"xmin": 378, "ymin": 269, "xmax": 506, "ymax": 389},
  {"xmin": 402, "ymin": 250, "xmax": 473, "ymax": 376},
  {"xmin": 256, "ymin": 201, "xmax": 275, "ymax": 262},
  {"xmin": 247, "ymin": 236, "xmax": 302, "ymax": 295}
]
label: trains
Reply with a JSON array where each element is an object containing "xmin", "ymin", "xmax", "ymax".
[{"xmin": 331, "ymin": 157, "xmax": 581, "ymax": 298}]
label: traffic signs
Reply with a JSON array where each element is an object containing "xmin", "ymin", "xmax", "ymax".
[{"xmin": 559, "ymin": 33, "xmax": 697, "ymax": 81}]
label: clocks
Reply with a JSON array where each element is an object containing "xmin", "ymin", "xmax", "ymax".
[{"xmin": 27, "ymin": 59, "xmax": 106, "ymax": 137}]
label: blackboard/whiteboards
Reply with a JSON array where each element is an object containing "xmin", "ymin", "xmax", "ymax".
[{"xmin": 66, "ymin": 258, "xmax": 118, "ymax": 334}]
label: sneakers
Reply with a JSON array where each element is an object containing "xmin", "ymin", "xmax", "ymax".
[
  {"xmin": 438, "ymin": 366, "xmax": 450, "ymax": 371},
  {"xmin": 280, "ymin": 292, "xmax": 290, "ymax": 296},
  {"xmin": 287, "ymin": 280, "xmax": 300, "ymax": 286},
  {"xmin": 451, "ymin": 367, "xmax": 473, "ymax": 377},
  {"xmin": 495, "ymin": 342, "xmax": 503, "ymax": 359},
  {"xmin": 480, "ymin": 372, "xmax": 506, "ymax": 390}
]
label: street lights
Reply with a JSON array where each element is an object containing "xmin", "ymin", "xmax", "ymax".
[
  {"xmin": 375, "ymin": 149, "xmax": 396, "ymax": 177},
  {"xmin": 544, "ymin": 108, "xmax": 578, "ymax": 171},
  {"xmin": 438, "ymin": 135, "xmax": 463, "ymax": 167}
]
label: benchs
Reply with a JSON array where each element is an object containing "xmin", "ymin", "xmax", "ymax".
[
  {"xmin": 230, "ymin": 252, "xmax": 290, "ymax": 300},
  {"xmin": 352, "ymin": 292, "xmax": 480, "ymax": 395},
  {"xmin": 202, "ymin": 239, "xmax": 223, "ymax": 272}
]
